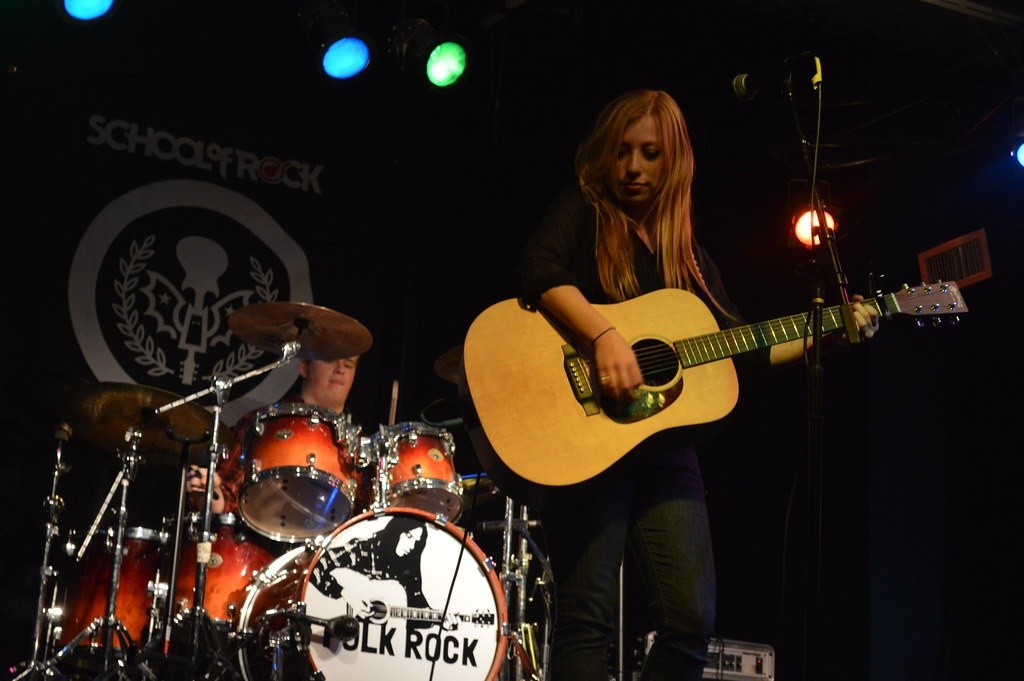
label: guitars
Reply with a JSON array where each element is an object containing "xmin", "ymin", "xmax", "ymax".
[{"xmin": 456, "ymin": 278, "xmax": 970, "ymax": 507}]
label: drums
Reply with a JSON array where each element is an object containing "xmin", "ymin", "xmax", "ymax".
[
  {"xmin": 234, "ymin": 401, "xmax": 367, "ymax": 544},
  {"xmin": 52, "ymin": 525, "xmax": 176, "ymax": 671},
  {"xmin": 370, "ymin": 420, "xmax": 464, "ymax": 525},
  {"xmin": 234, "ymin": 506, "xmax": 510, "ymax": 681},
  {"xmin": 157, "ymin": 511, "xmax": 282, "ymax": 642}
]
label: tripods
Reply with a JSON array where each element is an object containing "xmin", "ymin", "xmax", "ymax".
[{"xmin": 0, "ymin": 327, "xmax": 304, "ymax": 681}]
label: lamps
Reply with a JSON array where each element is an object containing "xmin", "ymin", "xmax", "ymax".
[
  {"xmin": 385, "ymin": 16, "xmax": 468, "ymax": 88},
  {"xmin": 785, "ymin": 178, "xmax": 837, "ymax": 249},
  {"xmin": 54, "ymin": 0, "xmax": 123, "ymax": 25},
  {"xmin": 297, "ymin": 0, "xmax": 377, "ymax": 84},
  {"xmin": 1008, "ymin": 96, "xmax": 1024, "ymax": 171}
]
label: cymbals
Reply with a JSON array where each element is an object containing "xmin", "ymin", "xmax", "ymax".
[
  {"xmin": 49, "ymin": 381, "xmax": 234, "ymax": 469},
  {"xmin": 227, "ymin": 302, "xmax": 374, "ymax": 361},
  {"xmin": 433, "ymin": 344, "xmax": 464, "ymax": 385}
]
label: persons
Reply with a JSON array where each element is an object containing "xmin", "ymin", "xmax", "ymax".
[
  {"xmin": 187, "ymin": 353, "xmax": 356, "ymax": 514},
  {"xmin": 520, "ymin": 89, "xmax": 879, "ymax": 681}
]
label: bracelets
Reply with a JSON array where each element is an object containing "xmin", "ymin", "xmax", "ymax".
[{"xmin": 591, "ymin": 327, "xmax": 616, "ymax": 346}]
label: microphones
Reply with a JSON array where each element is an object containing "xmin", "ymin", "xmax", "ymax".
[{"xmin": 730, "ymin": 52, "xmax": 814, "ymax": 101}]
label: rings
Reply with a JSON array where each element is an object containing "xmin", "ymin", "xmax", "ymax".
[
  {"xmin": 859, "ymin": 325, "xmax": 868, "ymax": 333},
  {"xmin": 600, "ymin": 375, "xmax": 609, "ymax": 382}
]
